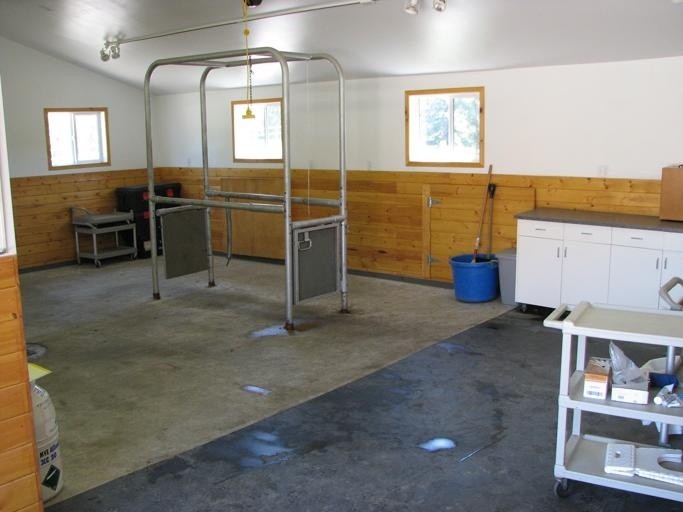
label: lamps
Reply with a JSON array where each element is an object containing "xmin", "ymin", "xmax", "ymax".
[
  {"xmin": 404, "ymin": 1, "xmax": 448, "ymax": 16},
  {"xmin": 100, "ymin": 40, "xmax": 121, "ymax": 62}
]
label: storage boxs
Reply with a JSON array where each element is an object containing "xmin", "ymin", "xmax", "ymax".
[{"xmin": 116, "ymin": 182, "xmax": 182, "ymax": 259}]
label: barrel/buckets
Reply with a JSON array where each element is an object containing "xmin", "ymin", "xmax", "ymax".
[
  {"xmin": 449, "ymin": 253, "xmax": 499, "ymax": 303},
  {"xmin": 29, "ymin": 380, "xmax": 64, "ymax": 502}
]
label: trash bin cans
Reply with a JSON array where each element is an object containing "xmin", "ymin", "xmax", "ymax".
[{"xmin": 494, "ymin": 248, "xmax": 527, "ymax": 307}]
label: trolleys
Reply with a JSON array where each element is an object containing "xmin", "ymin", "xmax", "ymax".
[
  {"xmin": 69, "ymin": 206, "xmax": 138, "ymax": 269},
  {"xmin": 543, "ymin": 277, "xmax": 683, "ymax": 504}
]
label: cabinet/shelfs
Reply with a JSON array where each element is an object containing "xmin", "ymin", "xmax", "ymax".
[
  {"xmin": 514, "ymin": 220, "xmax": 683, "ymax": 312},
  {"xmin": 543, "ymin": 302, "xmax": 682, "ymax": 503}
]
